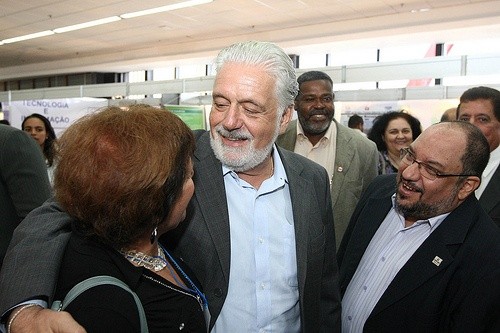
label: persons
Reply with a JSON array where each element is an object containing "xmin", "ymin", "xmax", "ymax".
[
  {"xmin": 336, "ymin": 121, "xmax": 500, "ymax": 333},
  {"xmin": 274, "ymin": 71, "xmax": 379, "ymax": 253},
  {"xmin": 0, "ymin": 102, "xmax": 59, "ymax": 268},
  {"xmin": 348, "ymin": 114, "xmax": 364, "ymax": 132},
  {"xmin": 0, "ymin": 41, "xmax": 342, "ymax": 333},
  {"xmin": 366, "ymin": 110, "xmax": 422, "ymax": 174},
  {"xmin": 455, "ymin": 86, "xmax": 500, "ymax": 230},
  {"xmin": 45, "ymin": 104, "xmax": 212, "ymax": 333},
  {"xmin": 440, "ymin": 107, "xmax": 456, "ymax": 121}
]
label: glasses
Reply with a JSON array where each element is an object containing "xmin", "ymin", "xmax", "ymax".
[{"xmin": 399, "ymin": 146, "xmax": 478, "ymax": 179}]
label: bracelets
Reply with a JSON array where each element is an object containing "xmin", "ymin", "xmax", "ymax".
[{"xmin": 7, "ymin": 304, "xmax": 37, "ymax": 333}]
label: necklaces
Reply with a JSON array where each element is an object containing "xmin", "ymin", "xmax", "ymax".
[{"xmin": 120, "ymin": 245, "xmax": 168, "ymax": 271}]
